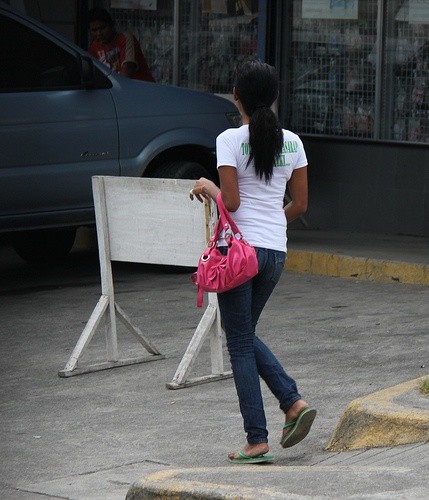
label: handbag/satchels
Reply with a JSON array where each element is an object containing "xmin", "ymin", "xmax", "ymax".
[{"xmin": 191, "ymin": 191, "xmax": 259, "ymax": 308}]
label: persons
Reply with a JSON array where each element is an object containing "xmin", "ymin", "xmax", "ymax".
[
  {"xmin": 88, "ymin": 8, "xmax": 155, "ymax": 83},
  {"xmin": 190, "ymin": 61, "xmax": 316, "ymax": 464}
]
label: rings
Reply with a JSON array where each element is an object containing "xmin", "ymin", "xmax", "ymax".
[{"xmin": 190, "ymin": 189, "xmax": 194, "ymax": 195}]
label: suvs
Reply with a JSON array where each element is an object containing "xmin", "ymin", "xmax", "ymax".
[{"xmin": 0, "ymin": 7, "xmax": 243, "ymax": 274}]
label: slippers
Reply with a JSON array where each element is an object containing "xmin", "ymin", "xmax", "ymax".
[
  {"xmin": 279, "ymin": 405, "xmax": 317, "ymax": 449},
  {"xmin": 227, "ymin": 449, "xmax": 275, "ymax": 464}
]
